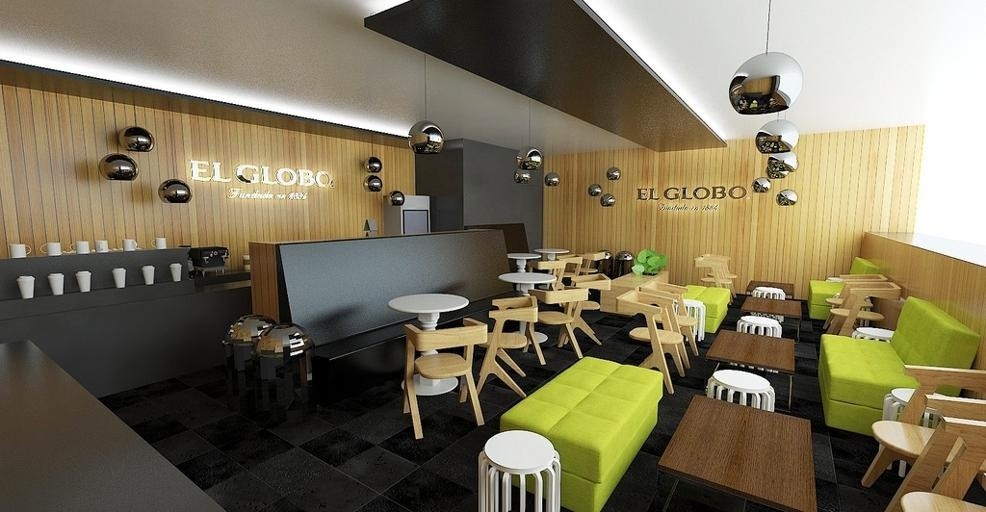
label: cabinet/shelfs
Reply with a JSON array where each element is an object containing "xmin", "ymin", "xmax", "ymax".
[
  {"xmin": 0, "ymin": 247, "xmax": 197, "ymax": 399},
  {"xmin": 249, "ymin": 227, "xmax": 515, "ymax": 388}
]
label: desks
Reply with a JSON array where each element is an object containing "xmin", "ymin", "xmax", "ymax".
[
  {"xmin": 745, "ymin": 280, "xmax": 794, "ymax": 301},
  {"xmin": 387, "ymin": 293, "xmax": 470, "ymax": 397},
  {"xmin": 507, "ymin": 253, "xmax": 541, "ymax": 292},
  {"xmin": 533, "ymin": 248, "xmax": 569, "ymax": 290},
  {"xmin": 657, "ymin": 394, "xmax": 817, "ymax": 512},
  {"xmin": 740, "ymin": 296, "xmax": 802, "ymax": 343},
  {"xmin": 498, "ymin": 272, "xmax": 558, "ymax": 345},
  {"xmin": 706, "ymin": 329, "xmax": 796, "ymax": 414}
]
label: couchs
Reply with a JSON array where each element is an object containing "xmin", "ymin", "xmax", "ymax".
[
  {"xmin": 500, "ymin": 356, "xmax": 664, "ymax": 512},
  {"xmin": 682, "ymin": 284, "xmax": 731, "ymax": 334},
  {"xmin": 817, "ymin": 295, "xmax": 980, "ymax": 435},
  {"xmin": 808, "ymin": 257, "xmax": 879, "ymax": 320}
]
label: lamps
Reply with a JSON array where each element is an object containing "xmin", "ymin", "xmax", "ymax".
[
  {"xmin": 776, "ymin": 189, "xmax": 799, "ymax": 207},
  {"xmin": 756, "ymin": 118, "xmax": 799, "ymax": 153},
  {"xmin": 607, "ymin": 167, "xmax": 621, "ymax": 181},
  {"xmin": 512, "ymin": 168, "xmax": 532, "ymax": 184},
  {"xmin": 545, "ymin": 172, "xmax": 560, "ymax": 186},
  {"xmin": 99, "ymin": 153, "xmax": 138, "ymax": 182},
  {"xmin": 588, "ymin": 183, "xmax": 602, "ymax": 196},
  {"xmin": 600, "ymin": 193, "xmax": 616, "ymax": 207},
  {"xmin": 729, "ymin": 2, "xmax": 804, "ymax": 115},
  {"xmin": 364, "ymin": 174, "xmax": 382, "ymax": 192},
  {"xmin": 515, "ymin": 99, "xmax": 542, "ymax": 170},
  {"xmin": 388, "ymin": 190, "xmax": 405, "ymax": 206},
  {"xmin": 408, "ymin": 56, "xmax": 444, "ymax": 154},
  {"xmin": 767, "ymin": 153, "xmax": 798, "ymax": 173},
  {"xmin": 118, "ymin": 125, "xmax": 154, "ymax": 152},
  {"xmin": 765, "ymin": 166, "xmax": 789, "ymax": 178},
  {"xmin": 158, "ymin": 178, "xmax": 192, "ymax": 205},
  {"xmin": 364, "ymin": 156, "xmax": 382, "ymax": 173},
  {"xmin": 751, "ymin": 177, "xmax": 771, "ymax": 193}
]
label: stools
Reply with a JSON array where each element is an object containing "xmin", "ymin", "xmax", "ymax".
[
  {"xmin": 729, "ymin": 316, "xmax": 782, "ymax": 374},
  {"xmin": 190, "ymin": 247, "xmax": 228, "ymax": 276},
  {"xmin": 851, "ymin": 326, "xmax": 894, "ymax": 343},
  {"xmin": 477, "ymin": 429, "xmax": 561, "ymax": 512},
  {"xmin": 878, "ymin": 387, "xmax": 947, "ymax": 477},
  {"xmin": 673, "ymin": 299, "xmax": 706, "ymax": 342},
  {"xmin": 749, "ymin": 286, "xmax": 785, "ymax": 324},
  {"xmin": 705, "ymin": 369, "xmax": 775, "ymax": 413}
]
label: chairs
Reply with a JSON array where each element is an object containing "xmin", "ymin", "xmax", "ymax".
[
  {"xmin": 861, "ymin": 365, "xmax": 986, "ymax": 512},
  {"xmin": 527, "ymin": 258, "xmax": 565, "ymax": 307},
  {"xmin": 477, "ymin": 295, "xmax": 538, "ymax": 400},
  {"xmin": 826, "ymin": 281, "xmax": 902, "ymax": 337},
  {"xmin": 552, "ymin": 283, "xmax": 601, "ymax": 335},
  {"xmin": 701, "ymin": 253, "xmax": 737, "ymax": 299},
  {"xmin": 564, "ymin": 273, "xmax": 611, "ymax": 346},
  {"xmin": 616, "ymin": 289, "xmax": 686, "ymax": 395},
  {"xmin": 557, "ymin": 254, "xmax": 583, "ymax": 287},
  {"xmin": 694, "ymin": 257, "xmax": 733, "ymax": 305},
  {"xmin": 823, "ymin": 274, "xmax": 888, "ymax": 334},
  {"xmin": 401, "ymin": 316, "xmax": 485, "ymax": 441},
  {"xmin": 573, "ymin": 250, "xmax": 606, "ymax": 276},
  {"xmin": 522, "ymin": 284, "xmax": 583, "ymax": 367},
  {"xmin": 900, "ymin": 415, "xmax": 985, "ymax": 512},
  {"xmin": 639, "ymin": 280, "xmax": 699, "ymax": 369}
]
